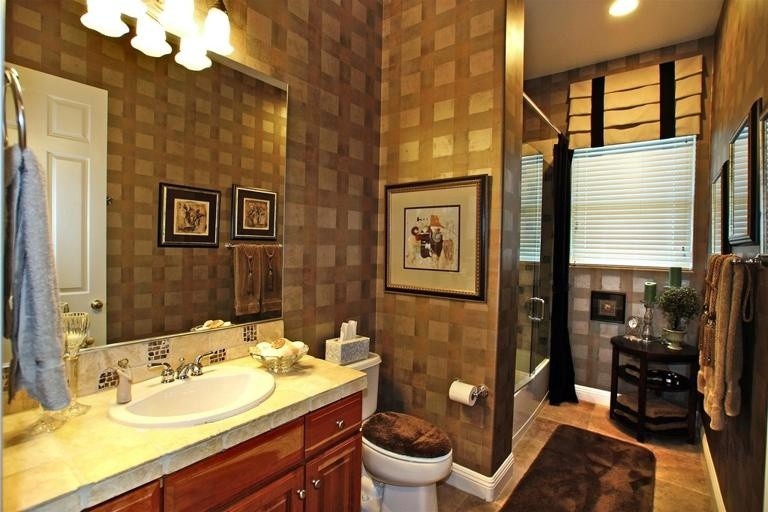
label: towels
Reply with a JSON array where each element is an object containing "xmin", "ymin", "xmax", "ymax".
[
  {"xmin": 233, "ymin": 244, "xmax": 260, "ymax": 315},
  {"xmin": 4, "ymin": 143, "xmax": 70, "ymax": 411},
  {"xmin": 261, "ymin": 244, "xmax": 282, "ymax": 313},
  {"xmin": 697, "ymin": 253, "xmax": 721, "ymax": 395},
  {"xmin": 703, "ymin": 254, "xmax": 754, "ymax": 430}
]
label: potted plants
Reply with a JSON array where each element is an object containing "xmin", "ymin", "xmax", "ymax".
[{"xmin": 656, "ymin": 285, "xmax": 701, "ymax": 351}]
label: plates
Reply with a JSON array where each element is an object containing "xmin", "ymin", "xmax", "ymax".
[{"xmin": 250, "ymin": 347, "xmax": 309, "ymax": 375}]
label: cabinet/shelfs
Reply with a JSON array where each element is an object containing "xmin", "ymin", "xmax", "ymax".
[
  {"xmin": 80, "ymin": 477, "xmax": 164, "ymax": 511},
  {"xmin": 305, "ymin": 389, "xmax": 362, "ymax": 512},
  {"xmin": 608, "ymin": 335, "xmax": 700, "ymax": 444},
  {"xmin": 162, "ymin": 417, "xmax": 305, "ymax": 512}
]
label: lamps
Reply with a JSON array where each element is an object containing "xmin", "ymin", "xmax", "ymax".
[
  {"xmin": 130, "ymin": 16, "xmax": 174, "ymax": 58},
  {"xmin": 174, "ymin": 36, "xmax": 212, "ymax": 72},
  {"xmin": 117, "ymin": 0, "xmax": 148, "ymax": 18},
  {"xmin": 201, "ymin": 8, "xmax": 234, "ymax": 56},
  {"xmin": 158, "ymin": 0, "xmax": 197, "ymax": 36},
  {"xmin": 79, "ymin": 0, "xmax": 130, "ymax": 39}
]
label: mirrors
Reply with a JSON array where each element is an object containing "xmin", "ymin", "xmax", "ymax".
[{"xmin": 4, "ymin": 0, "xmax": 291, "ymax": 363}]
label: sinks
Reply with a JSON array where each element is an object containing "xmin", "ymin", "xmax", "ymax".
[{"xmin": 107, "ymin": 365, "xmax": 276, "ymax": 428}]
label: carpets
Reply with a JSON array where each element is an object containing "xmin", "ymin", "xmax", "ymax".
[{"xmin": 499, "ymin": 424, "xmax": 656, "ymax": 512}]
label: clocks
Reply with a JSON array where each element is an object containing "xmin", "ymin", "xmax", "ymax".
[{"xmin": 622, "ymin": 316, "xmax": 643, "ymax": 343}]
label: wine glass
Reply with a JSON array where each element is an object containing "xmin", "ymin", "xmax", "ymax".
[{"xmin": 58, "ymin": 310, "xmax": 93, "ymax": 416}]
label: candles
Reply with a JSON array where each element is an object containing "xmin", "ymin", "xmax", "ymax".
[
  {"xmin": 669, "ymin": 267, "xmax": 682, "ymax": 288},
  {"xmin": 644, "ymin": 282, "xmax": 657, "ymax": 302}
]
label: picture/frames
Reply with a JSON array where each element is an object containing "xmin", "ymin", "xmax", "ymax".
[
  {"xmin": 590, "ymin": 290, "xmax": 626, "ymax": 325},
  {"xmin": 757, "ymin": 108, "xmax": 768, "ymax": 262},
  {"xmin": 230, "ymin": 184, "xmax": 279, "ymax": 240},
  {"xmin": 383, "ymin": 173, "xmax": 487, "ymax": 309},
  {"xmin": 727, "ymin": 97, "xmax": 764, "ymax": 247},
  {"xmin": 709, "ymin": 160, "xmax": 731, "ymax": 257},
  {"xmin": 157, "ymin": 181, "xmax": 223, "ymax": 249}
]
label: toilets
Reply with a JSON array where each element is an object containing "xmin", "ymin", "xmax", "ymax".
[{"xmin": 342, "ymin": 412, "xmax": 452, "ymax": 512}]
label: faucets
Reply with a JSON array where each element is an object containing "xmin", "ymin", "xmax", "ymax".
[{"xmin": 176, "ymin": 358, "xmax": 194, "ymax": 379}]
